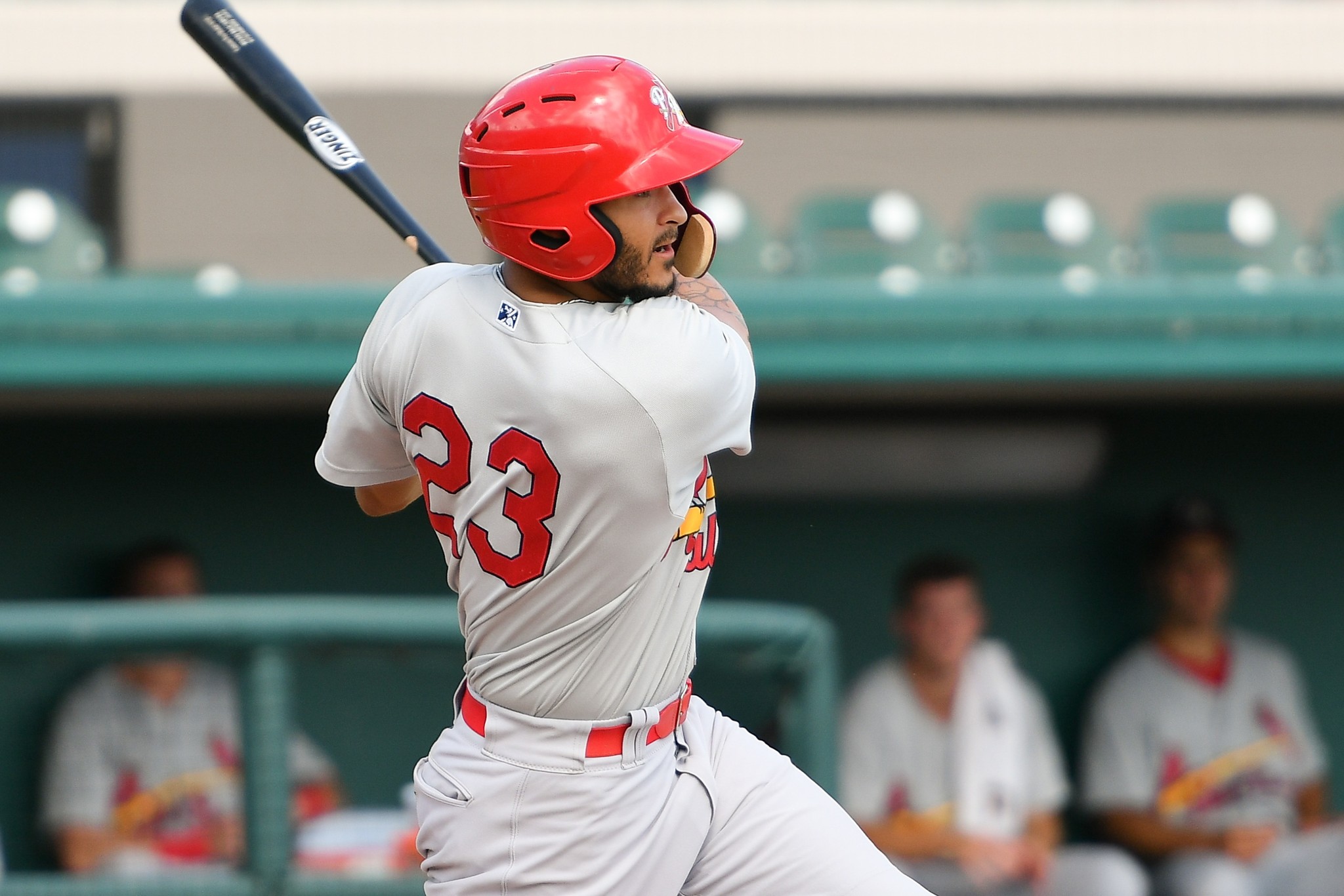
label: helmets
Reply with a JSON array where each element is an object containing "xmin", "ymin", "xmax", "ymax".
[{"xmin": 460, "ymin": 56, "xmax": 744, "ymax": 281}]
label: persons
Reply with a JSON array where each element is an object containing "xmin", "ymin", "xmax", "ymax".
[
  {"xmin": 315, "ymin": 57, "xmax": 938, "ymax": 896},
  {"xmin": 39, "ymin": 535, "xmax": 335, "ymax": 877},
  {"xmin": 841, "ymin": 555, "xmax": 1150, "ymax": 894},
  {"xmin": 1075, "ymin": 523, "xmax": 1344, "ymax": 896}
]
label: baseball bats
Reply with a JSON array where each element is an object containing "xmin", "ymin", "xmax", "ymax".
[{"xmin": 181, "ymin": 0, "xmax": 453, "ymax": 267}]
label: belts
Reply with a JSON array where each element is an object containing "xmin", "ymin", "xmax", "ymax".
[{"xmin": 457, "ymin": 682, "xmax": 694, "ymax": 758}]
